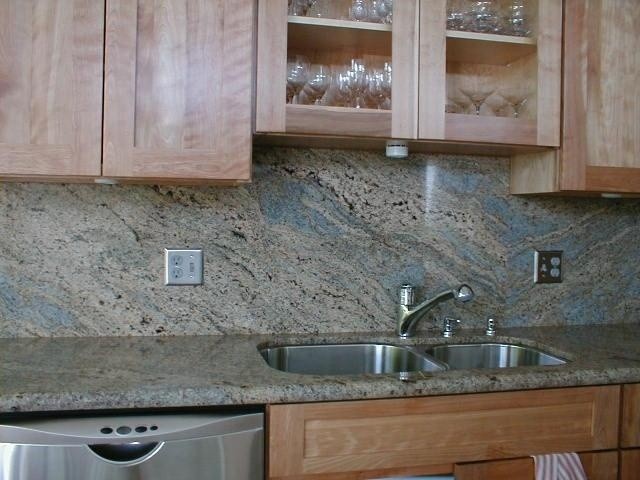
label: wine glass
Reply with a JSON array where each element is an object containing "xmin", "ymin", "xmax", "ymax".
[
  {"xmin": 446, "ymin": 76, "xmax": 535, "ymax": 117},
  {"xmin": 287, "ymin": 53, "xmax": 392, "ymax": 109}
]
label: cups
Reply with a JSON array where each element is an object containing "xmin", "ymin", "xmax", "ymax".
[
  {"xmin": 445, "ymin": 0, "xmax": 534, "ymax": 35},
  {"xmin": 288, "ymin": 1, "xmax": 393, "ymax": 24}
]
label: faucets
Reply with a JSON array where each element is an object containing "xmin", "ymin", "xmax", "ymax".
[{"xmin": 395, "ymin": 281, "xmax": 475, "ymax": 338}]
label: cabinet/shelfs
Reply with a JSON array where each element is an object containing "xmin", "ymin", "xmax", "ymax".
[
  {"xmin": 0, "ymin": 1, "xmax": 257, "ymax": 189},
  {"xmin": 256, "ymin": 1, "xmax": 562, "ymax": 156},
  {"xmin": 507, "ymin": 1, "xmax": 640, "ymax": 200},
  {"xmin": 263, "ymin": 382, "xmax": 622, "ymax": 479},
  {"xmin": 620, "ymin": 383, "xmax": 639, "ymax": 479}
]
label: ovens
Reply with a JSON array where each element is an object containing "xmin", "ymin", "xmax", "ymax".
[{"xmin": 0, "ymin": 409, "xmax": 265, "ymax": 480}]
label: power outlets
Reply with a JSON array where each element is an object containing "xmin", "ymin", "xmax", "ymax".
[
  {"xmin": 534, "ymin": 250, "xmax": 563, "ymax": 284},
  {"xmin": 161, "ymin": 248, "xmax": 205, "ymax": 287}
]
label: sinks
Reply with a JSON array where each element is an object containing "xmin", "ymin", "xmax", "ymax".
[
  {"xmin": 425, "ymin": 335, "xmax": 579, "ymax": 372},
  {"xmin": 256, "ymin": 339, "xmax": 448, "ymax": 377}
]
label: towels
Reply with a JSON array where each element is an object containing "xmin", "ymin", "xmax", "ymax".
[{"xmin": 532, "ymin": 452, "xmax": 589, "ymax": 480}]
